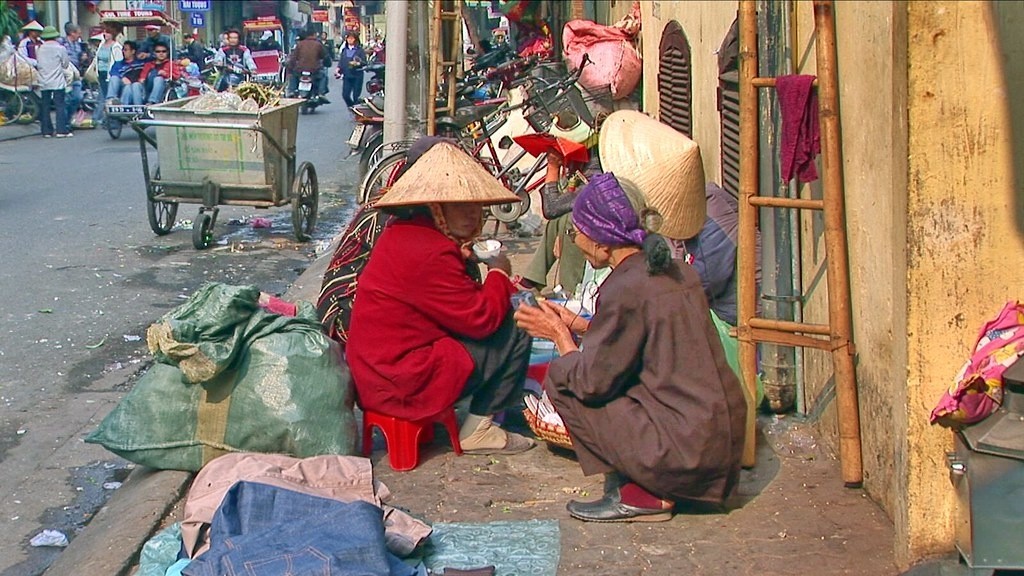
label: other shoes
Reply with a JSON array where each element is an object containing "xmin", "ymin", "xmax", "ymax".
[
  {"xmin": 318, "ymin": 94, "xmax": 331, "ymax": 105},
  {"xmin": 44, "ymin": 134, "xmax": 51, "ymax": 137},
  {"xmin": 56, "ymin": 132, "xmax": 74, "ymax": 137}
]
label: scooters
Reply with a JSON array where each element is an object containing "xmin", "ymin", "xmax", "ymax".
[
  {"xmin": 341, "ymin": 38, "xmax": 614, "ymax": 200},
  {"xmin": 213, "ymin": 64, "xmax": 253, "ymax": 92},
  {"xmin": 0, "ymin": 65, "xmax": 100, "ymax": 126}
]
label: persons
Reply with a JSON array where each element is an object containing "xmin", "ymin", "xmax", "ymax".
[
  {"xmin": 478, "ymin": 39, "xmax": 493, "ymax": 57},
  {"xmin": 335, "ymin": 30, "xmax": 367, "ymax": 121},
  {"xmin": 317, "ymin": 136, "xmax": 534, "ymax": 455},
  {"xmin": 17, "ymin": 20, "xmax": 333, "ymax": 136},
  {"xmin": 513, "ymin": 108, "xmax": 749, "ymax": 522}
]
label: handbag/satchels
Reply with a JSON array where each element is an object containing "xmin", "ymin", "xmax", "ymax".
[
  {"xmin": 142, "ymin": 69, "xmax": 157, "ymax": 103},
  {"xmin": 84, "ymin": 57, "xmax": 98, "ymax": 83},
  {"xmin": 709, "ymin": 308, "xmax": 763, "ymax": 407}
]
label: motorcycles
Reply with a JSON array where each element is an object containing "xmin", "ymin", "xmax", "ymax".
[{"xmin": 280, "ymin": 60, "xmax": 330, "ymax": 115}]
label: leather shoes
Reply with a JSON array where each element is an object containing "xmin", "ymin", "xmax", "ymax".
[{"xmin": 566, "ymin": 487, "xmax": 672, "ymax": 522}]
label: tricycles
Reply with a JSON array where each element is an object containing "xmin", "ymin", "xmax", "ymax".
[
  {"xmin": 241, "ymin": 15, "xmax": 288, "ymax": 86},
  {"xmin": 96, "ymin": 10, "xmax": 190, "ymax": 139}
]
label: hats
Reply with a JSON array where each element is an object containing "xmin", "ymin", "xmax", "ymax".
[
  {"xmin": 597, "ymin": 108, "xmax": 706, "ymax": 238},
  {"xmin": 39, "ymin": 25, "xmax": 60, "ymax": 38},
  {"xmin": 492, "ymin": 29, "xmax": 509, "ymax": 46},
  {"xmin": 21, "ymin": 20, "xmax": 43, "ymax": 30},
  {"xmin": 371, "ymin": 140, "xmax": 524, "ymax": 208},
  {"xmin": 90, "ymin": 32, "xmax": 104, "ymax": 41},
  {"xmin": 260, "ymin": 30, "xmax": 273, "ymax": 40},
  {"xmin": 144, "ymin": 24, "xmax": 162, "ymax": 31},
  {"xmin": 548, "ymin": 108, "xmax": 598, "ymax": 156},
  {"xmin": 364, "ymin": 96, "xmax": 392, "ymax": 115}
]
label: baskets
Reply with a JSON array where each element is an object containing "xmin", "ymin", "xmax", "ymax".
[
  {"xmin": 525, "ymin": 86, "xmax": 597, "ymax": 134},
  {"xmin": 531, "ymin": 63, "xmax": 568, "ymax": 84},
  {"xmin": 581, "ymin": 83, "xmax": 616, "ymax": 114}
]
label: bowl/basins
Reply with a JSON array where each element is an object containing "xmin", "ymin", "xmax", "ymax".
[{"xmin": 473, "ymin": 239, "xmax": 502, "ymax": 263}]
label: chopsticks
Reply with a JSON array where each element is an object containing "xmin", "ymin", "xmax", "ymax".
[{"xmin": 473, "ymin": 238, "xmax": 489, "ymax": 253}]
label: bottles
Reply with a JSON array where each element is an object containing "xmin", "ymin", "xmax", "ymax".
[{"xmin": 553, "ymin": 285, "xmax": 587, "ymax": 317}]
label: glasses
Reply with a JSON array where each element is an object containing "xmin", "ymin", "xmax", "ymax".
[
  {"xmin": 562, "ymin": 219, "xmax": 586, "ymax": 245},
  {"xmin": 497, "ymin": 35, "xmax": 504, "ymax": 40},
  {"xmin": 154, "ymin": 50, "xmax": 167, "ymax": 54},
  {"xmin": 121, "ymin": 48, "xmax": 133, "ymax": 52}
]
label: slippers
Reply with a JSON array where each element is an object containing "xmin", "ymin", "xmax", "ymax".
[{"xmin": 458, "ymin": 415, "xmax": 536, "ymax": 455}]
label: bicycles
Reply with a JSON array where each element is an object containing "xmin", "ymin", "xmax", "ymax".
[{"xmin": 357, "ymin": 51, "xmax": 600, "ymax": 247}]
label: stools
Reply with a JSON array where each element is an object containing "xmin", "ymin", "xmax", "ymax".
[{"xmin": 361, "ymin": 402, "xmax": 464, "ymax": 471}]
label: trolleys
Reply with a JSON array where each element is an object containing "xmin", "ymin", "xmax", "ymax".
[{"xmin": 128, "ymin": 119, "xmax": 320, "ymax": 251}]
